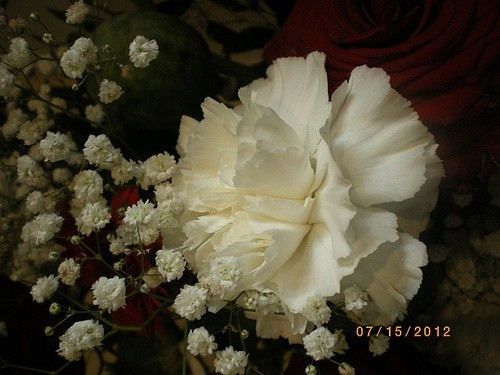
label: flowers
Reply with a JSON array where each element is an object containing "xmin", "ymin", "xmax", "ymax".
[{"xmin": 0, "ymin": 0, "xmax": 500, "ymax": 375}]
